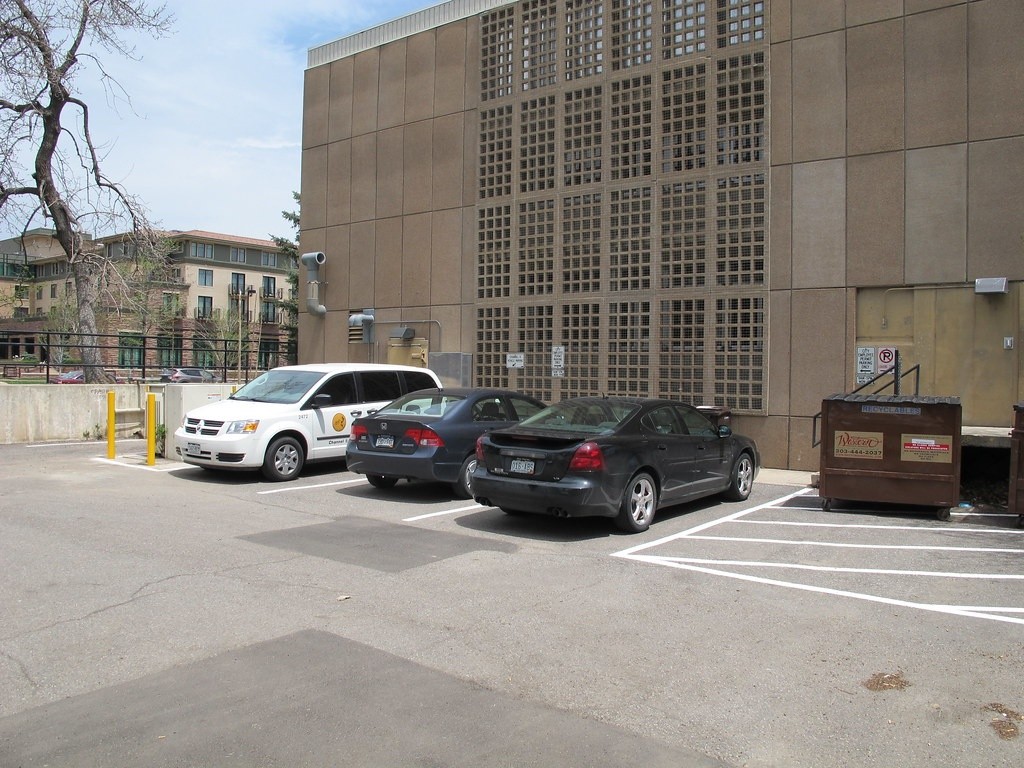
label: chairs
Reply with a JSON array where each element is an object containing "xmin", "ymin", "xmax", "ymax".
[
  {"xmin": 584, "ymin": 414, "xmax": 607, "ymax": 427},
  {"xmin": 478, "ymin": 402, "xmax": 506, "ymax": 420},
  {"xmin": 406, "ymin": 404, "xmax": 421, "ymax": 415}
]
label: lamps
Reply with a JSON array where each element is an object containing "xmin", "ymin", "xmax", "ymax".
[
  {"xmin": 389, "ymin": 327, "xmax": 416, "ymax": 340},
  {"xmin": 974, "ymin": 278, "xmax": 1011, "ymax": 295}
]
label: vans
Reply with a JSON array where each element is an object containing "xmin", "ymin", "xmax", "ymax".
[{"xmin": 172, "ymin": 363, "xmax": 447, "ymax": 483}]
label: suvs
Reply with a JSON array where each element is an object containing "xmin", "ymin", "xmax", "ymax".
[{"xmin": 160, "ymin": 367, "xmax": 223, "ymax": 383}]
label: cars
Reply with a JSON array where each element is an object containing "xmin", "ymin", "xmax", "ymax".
[
  {"xmin": 468, "ymin": 395, "xmax": 762, "ymax": 533},
  {"xmin": 49, "ymin": 370, "xmax": 126, "ymax": 385},
  {"xmin": 346, "ymin": 389, "xmax": 548, "ymax": 488}
]
